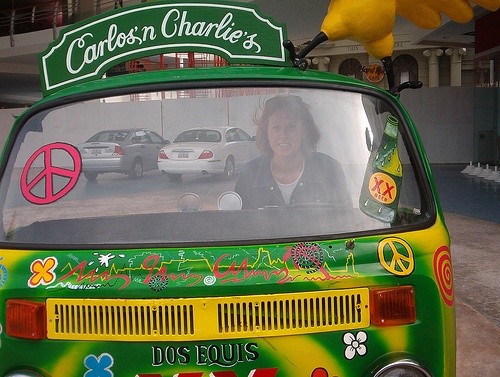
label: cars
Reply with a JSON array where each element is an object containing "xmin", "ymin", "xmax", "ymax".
[
  {"xmin": 158, "ymin": 129, "xmax": 264, "ymax": 183},
  {"xmin": 74, "ymin": 128, "xmax": 171, "ymax": 179},
  {"xmin": 0, "ymin": 0, "xmax": 458, "ymax": 377}
]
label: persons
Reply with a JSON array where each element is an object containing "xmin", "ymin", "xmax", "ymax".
[{"xmin": 235, "ymin": 96, "xmax": 353, "ymax": 210}]
